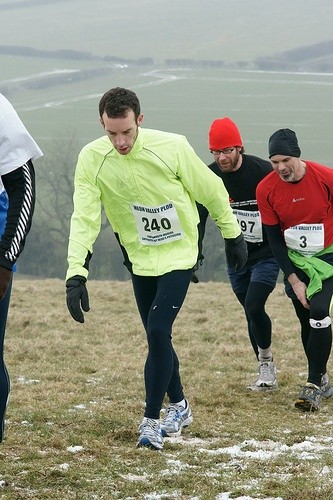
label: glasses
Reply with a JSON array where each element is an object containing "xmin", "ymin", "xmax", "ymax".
[{"xmin": 210, "ymin": 147, "xmax": 236, "ymax": 155}]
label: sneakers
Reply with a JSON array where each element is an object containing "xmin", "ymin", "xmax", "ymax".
[
  {"xmin": 246, "ymin": 383, "xmax": 279, "ymax": 392},
  {"xmin": 294, "ymin": 382, "xmax": 324, "ymax": 413},
  {"xmin": 255, "ymin": 361, "xmax": 276, "ymax": 386},
  {"xmin": 135, "ymin": 417, "xmax": 164, "ymax": 449},
  {"xmin": 161, "ymin": 398, "xmax": 194, "ymax": 437},
  {"xmin": 319, "ymin": 372, "xmax": 333, "ymax": 398}
]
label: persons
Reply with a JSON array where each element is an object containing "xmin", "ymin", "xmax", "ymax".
[
  {"xmin": 190, "ymin": 117, "xmax": 281, "ymax": 393},
  {"xmin": 65, "ymin": 86, "xmax": 248, "ymax": 452},
  {"xmin": 0, "ymin": 92, "xmax": 44, "ymax": 445},
  {"xmin": 256, "ymin": 128, "xmax": 333, "ymax": 413}
]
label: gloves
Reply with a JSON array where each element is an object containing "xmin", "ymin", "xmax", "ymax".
[
  {"xmin": 66, "ymin": 275, "xmax": 91, "ymax": 323},
  {"xmin": 224, "ymin": 231, "xmax": 248, "ymax": 274},
  {"xmin": 0, "ymin": 266, "xmax": 14, "ymax": 300},
  {"xmin": 189, "ymin": 260, "xmax": 199, "ymax": 284}
]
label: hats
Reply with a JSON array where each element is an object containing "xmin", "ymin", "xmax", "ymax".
[
  {"xmin": 209, "ymin": 117, "xmax": 242, "ymax": 150},
  {"xmin": 269, "ymin": 129, "xmax": 301, "ymax": 159}
]
